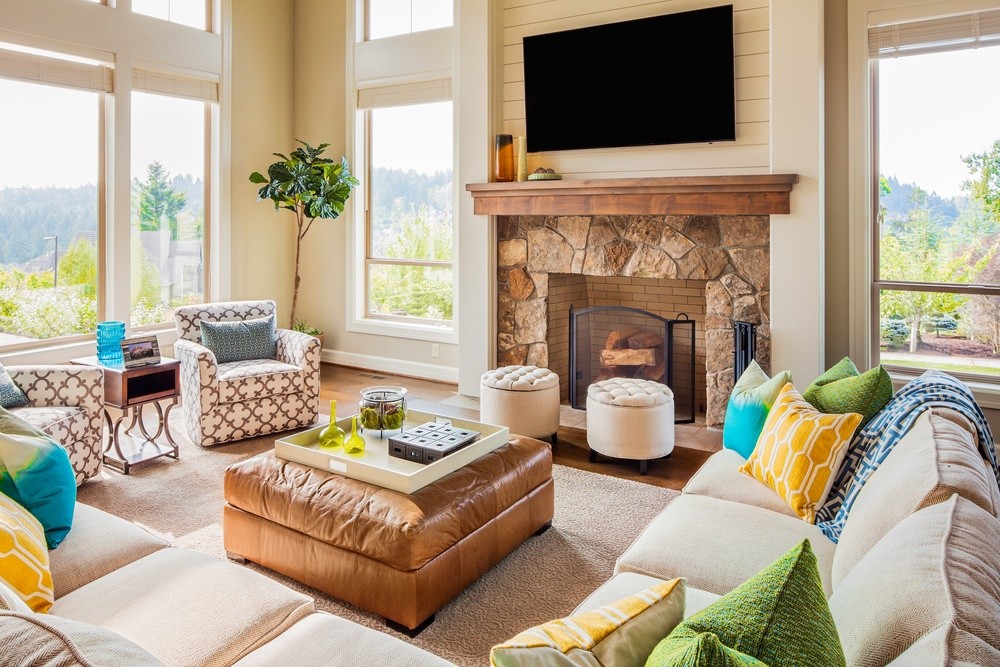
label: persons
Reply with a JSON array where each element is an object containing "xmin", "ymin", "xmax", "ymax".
[{"xmin": 131, "ymin": 347, "xmax": 143, "ymax": 359}]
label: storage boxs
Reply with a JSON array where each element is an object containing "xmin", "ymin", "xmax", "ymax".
[{"xmin": 275, "ymin": 407, "xmax": 509, "ymax": 494}]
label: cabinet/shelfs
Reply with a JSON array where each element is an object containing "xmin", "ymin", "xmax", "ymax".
[{"xmin": 70, "ymin": 356, "xmax": 181, "ymax": 474}]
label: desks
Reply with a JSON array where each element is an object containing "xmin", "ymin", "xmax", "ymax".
[{"xmin": 223, "ymin": 432, "xmax": 554, "ymax": 638}]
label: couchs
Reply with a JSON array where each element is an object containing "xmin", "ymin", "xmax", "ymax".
[
  {"xmin": 4, "ymin": 364, "xmax": 103, "ymax": 488},
  {"xmin": 0, "ymin": 502, "xmax": 461, "ymax": 667},
  {"xmin": 173, "ymin": 299, "xmax": 321, "ymax": 447},
  {"xmin": 569, "ymin": 370, "xmax": 1000, "ymax": 667}
]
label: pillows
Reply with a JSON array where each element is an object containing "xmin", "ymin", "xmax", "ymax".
[
  {"xmin": 0, "ymin": 363, "xmax": 30, "ymax": 408},
  {"xmin": 0, "ymin": 491, "xmax": 54, "ymax": 615},
  {"xmin": 199, "ymin": 314, "xmax": 275, "ymax": 362},
  {"xmin": 645, "ymin": 536, "xmax": 849, "ymax": 667},
  {"xmin": 0, "ymin": 406, "xmax": 78, "ymax": 549},
  {"xmin": 489, "ymin": 577, "xmax": 687, "ymax": 667},
  {"xmin": 719, "ymin": 356, "xmax": 893, "ymax": 525}
]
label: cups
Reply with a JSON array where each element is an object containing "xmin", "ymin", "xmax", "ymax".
[
  {"xmin": 494, "ymin": 134, "xmax": 514, "ymax": 182},
  {"xmin": 96, "ymin": 321, "xmax": 125, "ymax": 360}
]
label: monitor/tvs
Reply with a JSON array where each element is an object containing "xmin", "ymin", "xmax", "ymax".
[{"xmin": 522, "ymin": 4, "xmax": 738, "ymax": 153}]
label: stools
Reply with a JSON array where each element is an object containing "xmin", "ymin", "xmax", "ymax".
[
  {"xmin": 480, "ymin": 364, "xmax": 559, "ymax": 454},
  {"xmin": 585, "ymin": 376, "xmax": 675, "ymax": 474}
]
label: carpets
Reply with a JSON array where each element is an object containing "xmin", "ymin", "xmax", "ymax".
[{"xmin": 76, "ymin": 414, "xmax": 680, "ymax": 667}]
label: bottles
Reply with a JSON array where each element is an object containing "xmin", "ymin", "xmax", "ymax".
[
  {"xmin": 318, "ymin": 399, "xmax": 346, "ymax": 448},
  {"xmin": 344, "ymin": 416, "xmax": 366, "ymax": 454}
]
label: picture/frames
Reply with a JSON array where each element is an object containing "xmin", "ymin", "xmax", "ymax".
[{"xmin": 121, "ymin": 334, "xmax": 161, "ymax": 368}]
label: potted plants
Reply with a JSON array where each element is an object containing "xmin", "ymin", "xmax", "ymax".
[{"xmin": 247, "ymin": 138, "xmax": 360, "ymax": 351}]
